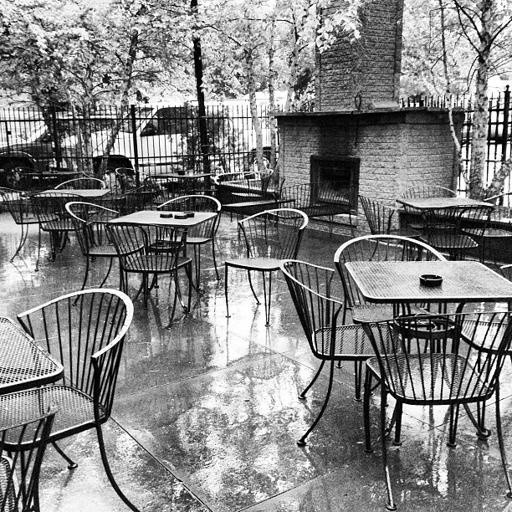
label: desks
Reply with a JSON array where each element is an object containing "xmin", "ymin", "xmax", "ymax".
[
  {"xmin": 394, "ymin": 198, "xmax": 491, "ymax": 262},
  {"xmin": 344, "ymin": 261, "xmax": 512, "ymax": 445},
  {"xmin": 0, "ymin": 316, "xmax": 64, "ymax": 512}
]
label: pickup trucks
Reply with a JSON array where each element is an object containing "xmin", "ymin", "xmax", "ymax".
[{"xmin": 62, "ymin": 106, "xmax": 294, "ymax": 180}]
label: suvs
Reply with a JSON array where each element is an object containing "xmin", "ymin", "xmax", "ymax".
[{"xmin": 1, "ymin": 117, "xmax": 113, "ymax": 189}]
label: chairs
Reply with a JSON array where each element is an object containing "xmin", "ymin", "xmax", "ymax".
[
  {"xmin": 334, "ymin": 234, "xmax": 449, "ymax": 368},
  {"xmin": 279, "ymin": 259, "xmax": 404, "ymax": 446},
  {"xmin": 358, "ymin": 195, "xmax": 422, "ymax": 260},
  {"xmin": 444, "ymin": 265, "xmax": 512, "ymax": 448},
  {"xmin": 352, "ymin": 312, "xmax": 512, "ymax": 511},
  {"xmin": 0, "ymin": 288, "xmax": 140, "ymax": 512},
  {"xmin": 265, "ymin": 182, "xmax": 355, "ymax": 245},
  {"xmin": 401, "ymin": 185, "xmax": 459, "ymax": 262},
  {"xmin": 460, "ymin": 192, "xmax": 512, "ymax": 263},
  {"xmin": 1, "ymin": 167, "xmax": 223, "ymax": 326},
  {"xmin": 225, "ymin": 207, "xmax": 309, "ymax": 328},
  {"xmin": 419, "ymin": 206, "xmax": 495, "ymax": 261},
  {"xmin": 0, "ymin": 401, "xmax": 59, "ymax": 512}
]
